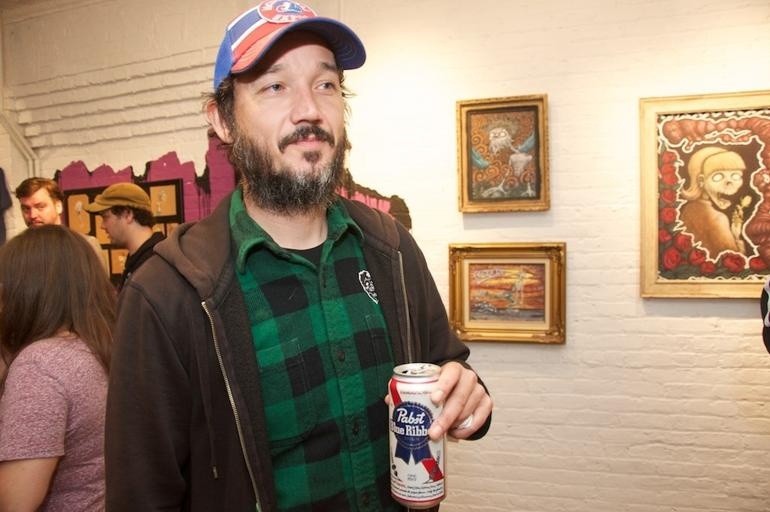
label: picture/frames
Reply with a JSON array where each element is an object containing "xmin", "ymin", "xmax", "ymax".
[
  {"xmin": 444, "ymin": 239, "xmax": 569, "ymax": 345},
  {"xmin": 636, "ymin": 88, "xmax": 770, "ymax": 305},
  {"xmin": 453, "ymin": 94, "xmax": 553, "ymax": 216}
]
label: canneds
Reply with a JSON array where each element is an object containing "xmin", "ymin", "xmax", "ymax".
[{"xmin": 387, "ymin": 363, "xmax": 447, "ymax": 509}]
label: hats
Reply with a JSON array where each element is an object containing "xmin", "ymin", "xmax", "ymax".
[
  {"xmin": 210, "ymin": 0, "xmax": 366, "ymax": 98},
  {"xmin": 83, "ymin": 182, "xmax": 155, "ymax": 216}
]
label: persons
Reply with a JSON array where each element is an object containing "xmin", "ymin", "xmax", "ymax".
[
  {"xmin": 105, "ymin": 0, "xmax": 494, "ymax": 512},
  {"xmin": 680, "ymin": 145, "xmax": 746, "ymax": 258},
  {"xmin": 760, "ymin": 282, "xmax": 770, "ymax": 352}
]
